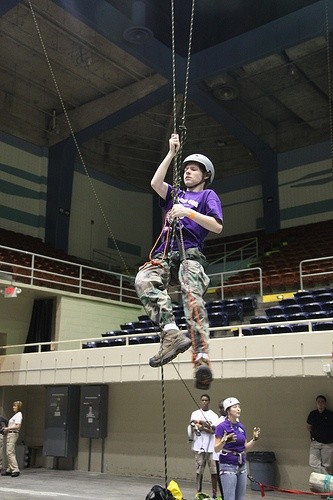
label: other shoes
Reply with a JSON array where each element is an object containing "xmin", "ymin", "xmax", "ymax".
[
  {"xmin": 9, "ymin": 472, "xmax": 20, "ymax": 477},
  {"xmin": 1, "ymin": 470, "xmax": 10, "ymax": 476}
]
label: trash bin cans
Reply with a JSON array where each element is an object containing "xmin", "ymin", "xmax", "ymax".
[{"xmin": 247, "ymin": 451, "xmax": 274, "ymax": 491}]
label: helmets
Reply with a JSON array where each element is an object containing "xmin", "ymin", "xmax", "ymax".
[
  {"xmin": 180, "ymin": 152, "xmax": 214, "ymax": 186},
  {"xmin": 222, "ymin": 397, "xmax": 242, "ymax": 411}
]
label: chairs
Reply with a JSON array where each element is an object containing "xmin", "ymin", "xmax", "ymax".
[
  {"xmin": 217, "ymin": 245, "xmax": 333, "ymax": 298},
  {"xmin": 82, "ymin": 297, "xmax": 257, "ymax": 349},
  {"xmin": 0, "ymin": 250, "xmax": 130, "ymax": 287},
  {"xmin": 233, "ymin": 288, "xmax": 333, "ymax": 336}
]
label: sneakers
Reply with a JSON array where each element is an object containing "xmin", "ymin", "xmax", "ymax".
[
  {"xmin": 192, "ymin": 359, "xmax": 214, "ymax": 389},
  {"xmin": 147, "ymin": 328, "xmax": 193, "ymax": 369}
]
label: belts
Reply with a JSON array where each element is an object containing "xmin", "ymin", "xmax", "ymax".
[{"xmin": 7, "ymin": 431, "xmax": 19, "ymax": 433}]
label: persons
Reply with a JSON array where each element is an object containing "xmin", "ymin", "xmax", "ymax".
[
  {"xmin": 134, "ymin": 134, "xmax": 223, "ymax": 390},
  {"xmin": 306, "ymin": 395, "xmax": 333, "ymax": 475},
  {"xmin": 0, "ymin": 416, "xmax": 8, "ymax": 472},
  {"xmin": 211, "ymin": 397, "xmax": 260, "ymax": 500},
  {"xmin": 191, "ymin": 393, "xmax": 219, "ymax": 499},
  {"xmin": 2, "ymin": 401, "xmax": 22, "ymax": 478}
]
